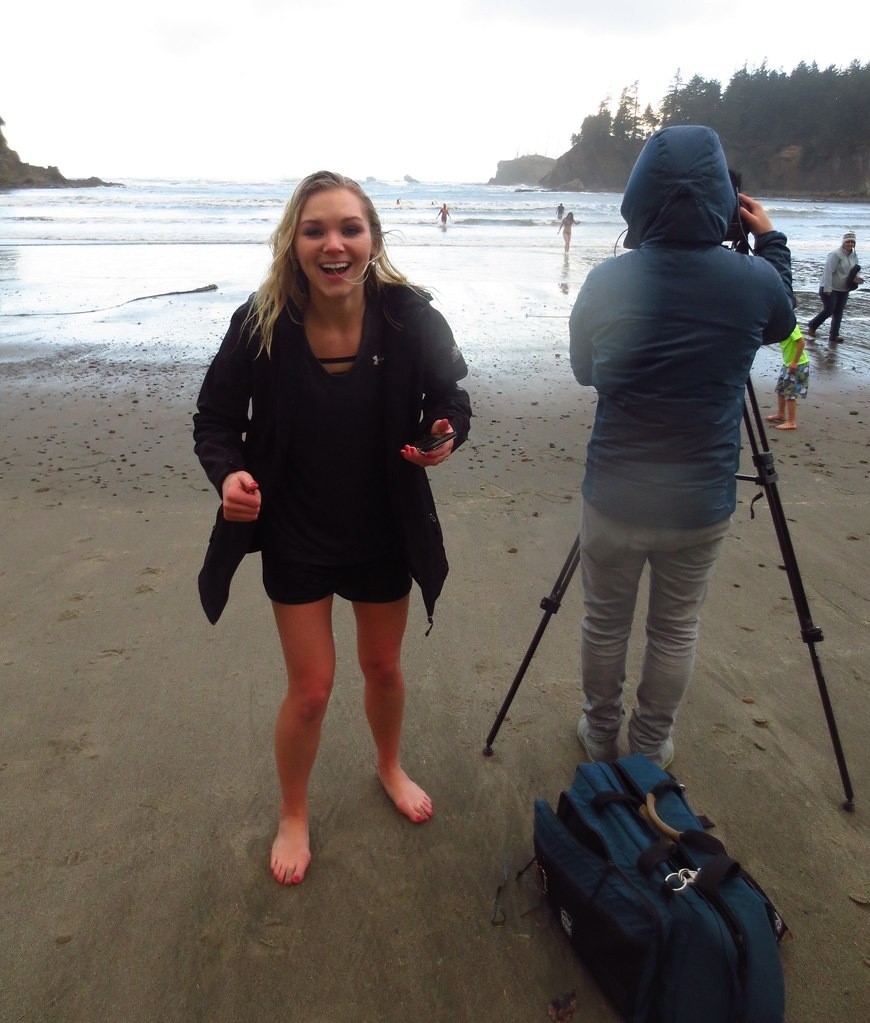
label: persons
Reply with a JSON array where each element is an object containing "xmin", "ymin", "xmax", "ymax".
[
  {"xmin": 556, "ymin": 212, "xmax": 580, "ymax": 252},
  {"xmin": 569, "ymin": 126, "xmax": 796, "ymax": 772},
  {"xmin": 808, "ymin": 232, "xmax": 864, "ymax": 341},
  {"xmin": 431, "ymin": 202, "xmax": 434, "ymax": 205},
  {"xmin": 194, "ymin": 172, "xmax": 473, "ymax": 891},
  {"xmin": 763, "ymin": 324, "xmax": 810, "ymax": 431},
  {"xmin": 556, "ymin": 203, "xmax": 565, "ymax": 220},
  {"xmin": 436, "ymin": 203, "xmax": 451, "ymax": 226},
  {"xmin": 396, "ymin": 197, "xmax": 400, "ymax": 205}
]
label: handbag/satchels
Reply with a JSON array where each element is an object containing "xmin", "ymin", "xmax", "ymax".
[{"xmin": 531, "ymin": 752, "xmax": 786, "ymax": 1023}]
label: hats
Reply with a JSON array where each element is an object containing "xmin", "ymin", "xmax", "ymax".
[{"xmin": 841, "ymin": 229, "xmax": 856, "ymax": 256}]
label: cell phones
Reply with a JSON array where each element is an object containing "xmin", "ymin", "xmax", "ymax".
[{"xmin": 412, "ymin": 431, "xmax": 459, "ymax": 451}]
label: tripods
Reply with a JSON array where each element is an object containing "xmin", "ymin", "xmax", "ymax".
[{"xmin": 485, "ymin": 375, "xmax": 854, "ymax": 797}]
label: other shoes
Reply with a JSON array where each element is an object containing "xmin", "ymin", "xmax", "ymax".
[
  {"xmin": 660, "ymin": 738, "xmax": 674, "ymax": 770},
  {"xmin": 577, "ymin": 715, "xmax": 598, "ymax": 763},
  {"xmin": 833, "ymin": 335, "xmax": 843, "ymax": 341},
  {"xmin": 808, "ymin": 326, "xmax": 815, "ymax": 336}
]
